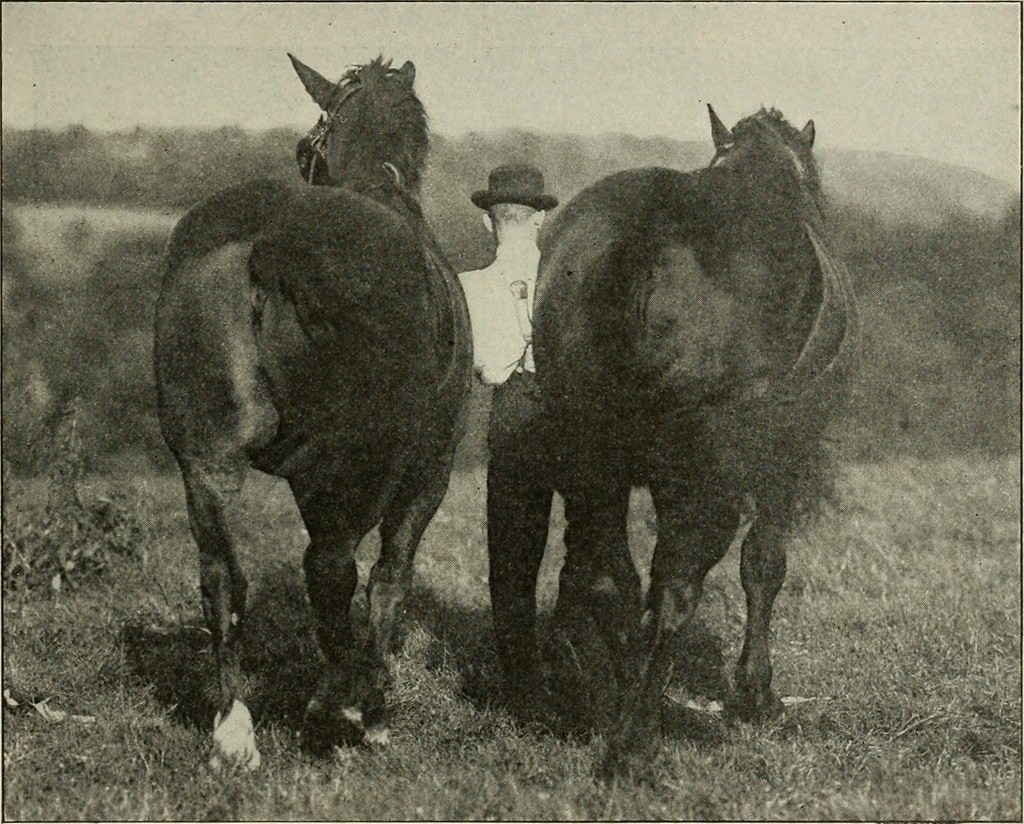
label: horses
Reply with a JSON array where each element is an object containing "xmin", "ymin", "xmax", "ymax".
[{"xmin": 152, "ymin": 52, "xmax": 859, "ymax": 789}]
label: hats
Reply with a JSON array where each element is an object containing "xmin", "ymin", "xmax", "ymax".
[{"xmin": 470, "ymin": 164, "xmax": 558, "ymax": 212}]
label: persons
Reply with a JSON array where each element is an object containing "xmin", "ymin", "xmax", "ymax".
[{"xmin": 458, "ymin": 164, "xmax": 603, "ymax": 722}]
